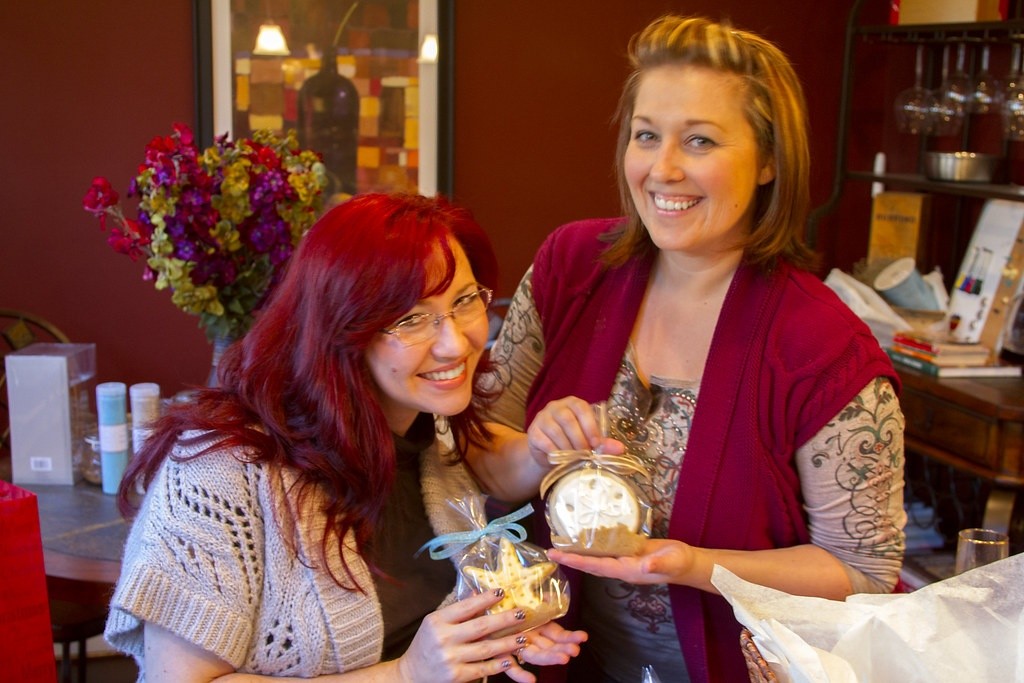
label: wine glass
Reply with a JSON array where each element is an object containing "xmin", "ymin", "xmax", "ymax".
[{"xmin": 892, "ymin": 33, "xmax": 1024, "ymax": 142}]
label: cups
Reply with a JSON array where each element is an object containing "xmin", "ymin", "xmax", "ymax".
[
  {"xmin": 873, "ymin": 257, "xmax": 939, "ymax": 315},
  {"xmin": 955, "ymin": 528, "xmax": 1010, "ymax": 575}
]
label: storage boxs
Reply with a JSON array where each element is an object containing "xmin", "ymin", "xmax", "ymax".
[{"xmin": 5, "ymin": 342, "xmax": 97, "ymax": 486}]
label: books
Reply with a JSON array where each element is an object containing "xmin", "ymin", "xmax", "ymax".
[{"xmin": 882, "ymin": 198, "xmax": 1024, "ymax": 378}]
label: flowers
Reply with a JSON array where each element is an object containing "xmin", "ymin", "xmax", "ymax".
[{"xmin": 82, "ymin": 122, "xmax": 327, "ymax": 344}]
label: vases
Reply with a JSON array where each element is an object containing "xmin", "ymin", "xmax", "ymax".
[{"xmin": 206, "ymin": 337, "xmax": 236, "ymax": 389}]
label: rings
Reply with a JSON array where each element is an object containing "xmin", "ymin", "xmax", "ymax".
[{"xmin": 517, "ymin": 643, "xmax": 530, "ymax": 664}]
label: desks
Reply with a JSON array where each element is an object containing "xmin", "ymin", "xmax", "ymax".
[
  {"xmin": 15, "ymin": 483, "xmax": 144, "ymax": 581},
  {"xmin": 894, "ymin": 358, "xmax": 1024, "ymax": 535}
]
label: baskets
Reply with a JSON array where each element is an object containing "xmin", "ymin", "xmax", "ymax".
[{"xmin": 738, "ymin": 626, "xmax": 844, "ymax": 683}]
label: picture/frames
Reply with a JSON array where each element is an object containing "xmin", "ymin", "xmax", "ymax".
[{"xmin": 191, "ymin": 1, "xmax": 455, "ymax": 206}]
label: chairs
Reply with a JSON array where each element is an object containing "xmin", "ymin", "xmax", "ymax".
[{"xmin": 0, "ymin": 307, "xmax": 117, "ymax": 682}]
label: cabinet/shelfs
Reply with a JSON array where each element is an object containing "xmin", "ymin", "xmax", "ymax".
[{"xmin": 805, "ymin": 0, "xmax": 1023, "ymax": 249}]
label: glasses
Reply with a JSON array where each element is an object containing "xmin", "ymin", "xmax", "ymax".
[{"xmin": 379, "ymin": 283, "xmax": 493, "ymax": 345}]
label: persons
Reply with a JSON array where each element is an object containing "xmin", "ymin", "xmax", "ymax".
[
  {"xmin": 103, "ymin": 194, "xmax": 587, "ymax": 683},
  {"xmin": 454, "ymin": 18, "xmax": 907, "ymax": 683}
]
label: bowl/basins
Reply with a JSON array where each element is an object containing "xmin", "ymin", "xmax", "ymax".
[{"xmin": 924, "ymin": 152, "xmax": 1004, "ymax": 184}]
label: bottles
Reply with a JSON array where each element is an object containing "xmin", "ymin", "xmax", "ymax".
[
  {"xmin": 96, "ymin": 382, "xmax": 130, "ymax": 496},
  {"xmin": 130, "ymin": 382, "xmax": 162, "ymax": 495}
]
